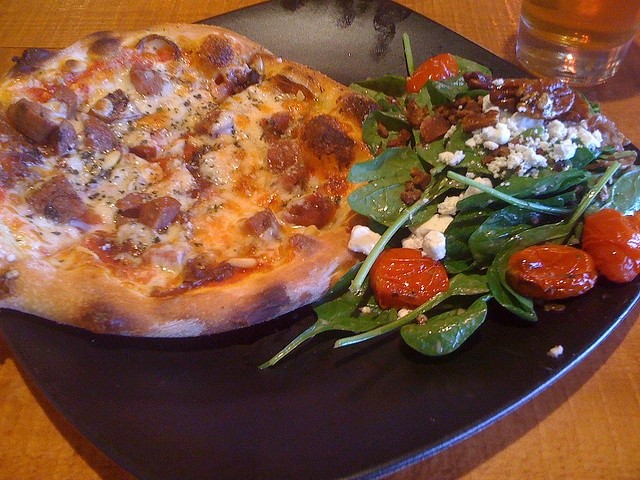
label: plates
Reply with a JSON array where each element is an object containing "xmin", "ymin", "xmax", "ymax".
[{"xmin": 0, "ymin": 1, "xmax": 640, "ymax": 480}]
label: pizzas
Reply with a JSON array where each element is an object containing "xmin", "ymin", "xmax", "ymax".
[{"xmin": 0, "ymin": 16, "xmax": 380, "ymax": 339}]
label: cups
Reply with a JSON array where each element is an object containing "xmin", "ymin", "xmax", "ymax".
[{"xmin": 517, "ymin": 1, "xmax": 640, "ymax": 88}]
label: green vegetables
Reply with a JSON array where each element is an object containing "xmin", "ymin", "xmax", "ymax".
[{"xmin": 256, "ymin": 36, "xmax": 640, "ymax": 371}]
